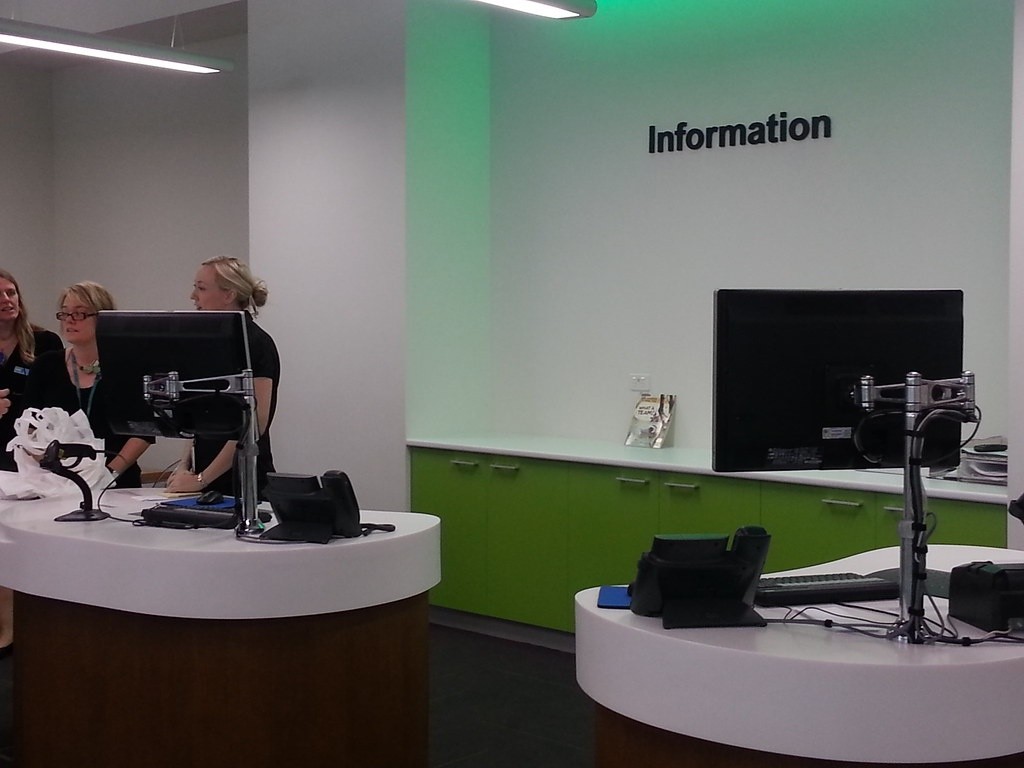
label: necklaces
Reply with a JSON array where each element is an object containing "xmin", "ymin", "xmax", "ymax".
[{"xmin": 70, "ymin": 348, "xmax": 100, "ymax": 374}]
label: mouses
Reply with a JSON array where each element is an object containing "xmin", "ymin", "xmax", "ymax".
[{"xmin": 197, "ymin": 490, "xmax": 224, "ymax": 506}]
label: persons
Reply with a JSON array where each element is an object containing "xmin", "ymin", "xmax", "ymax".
[
  {"xmin": 164, "ymin": 255, "xmax": 280, "ymax": 502},
  {"xmin": 0, "ymin": 269, "xmax": 64, "ymax": 473},
  {"xmin": 22, "ymin": 281, "xmax": 156, "ymax": 489}
]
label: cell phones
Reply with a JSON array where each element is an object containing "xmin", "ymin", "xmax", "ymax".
[{"xmin": 598, "ymin": 585, "xmax": 632, "ymax": 610}]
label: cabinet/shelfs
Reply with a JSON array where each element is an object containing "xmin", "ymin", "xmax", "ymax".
[{"xmin": 406, "ymin": 432, "xmax": 1008, "ymax": 654}]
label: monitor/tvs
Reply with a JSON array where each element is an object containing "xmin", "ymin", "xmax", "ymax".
[
  {"xmin": 96, "ymin": 310, "xmax": 260, "ymax": 443},
  {"xmin": 711, "ymin": 289, "xmax": 965, "ymax": 473}
]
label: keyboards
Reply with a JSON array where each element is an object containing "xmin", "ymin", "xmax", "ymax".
[
  {"xmin": 753, "ymin": 573, "xmax": 900, "ymax": 607},
  {"xmin": 175, "ymin": 506, "xmax": 272, "ymax": 522}
]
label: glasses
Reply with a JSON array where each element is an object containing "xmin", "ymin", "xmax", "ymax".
[{"xmin": 56, "ymin": 312, "xmax": 95, "ymax": 321}]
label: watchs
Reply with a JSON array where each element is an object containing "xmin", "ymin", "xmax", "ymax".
[{"xmin": 104, "ymin": 465, "xmax": 119, "ymax": 478}]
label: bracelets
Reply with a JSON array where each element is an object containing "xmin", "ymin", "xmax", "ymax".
[
  {"xmin": 117, "ymin": 454, "xmax": 131, "ymax": 468},
  {"xmin": 197, "ymin": 473, "xmax": 208, "ymax": 488}
]
